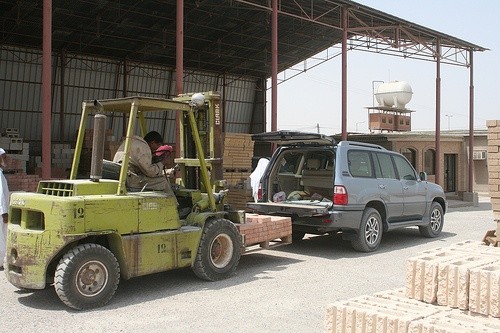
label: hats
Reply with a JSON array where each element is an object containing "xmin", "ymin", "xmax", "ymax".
[{"xmin": 0, "ymin": 148, "xmax": 5, "ymax": 155}]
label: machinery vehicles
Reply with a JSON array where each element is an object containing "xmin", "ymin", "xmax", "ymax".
[{"xmin": 5, "ymin": 91, "xmax": 247, "ymax": 312}]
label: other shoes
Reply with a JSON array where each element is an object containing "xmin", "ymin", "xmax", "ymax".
[{"xmin": 179, "ymin": 207, "xmax": 191, "ymax": 219}]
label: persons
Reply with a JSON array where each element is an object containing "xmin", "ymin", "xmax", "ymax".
[
  {"xmin": 0, "ymin": 147, "xmax": 10, "ymax": 271},
  {"xmin": 113, "ymin": 130, "xmax": 190, "ymax": 219}
]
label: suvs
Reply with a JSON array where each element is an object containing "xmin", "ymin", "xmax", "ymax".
[{"xmin": 246, "ymin": 130, "xmax": 448, "ymax": 252}]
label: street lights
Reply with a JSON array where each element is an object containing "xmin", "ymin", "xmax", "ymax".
[{"xmin": 445, "ymin": 114, "xmax": 453, "ymax": 130}]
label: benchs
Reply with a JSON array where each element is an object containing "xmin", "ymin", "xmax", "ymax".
[
  {"xmin": 102, "ymin": 159, "xmax": 139, "ymax": 191},
  {"xmin": 302, "ymin": 170, "xmax": 366, "ymax": 200}
]
